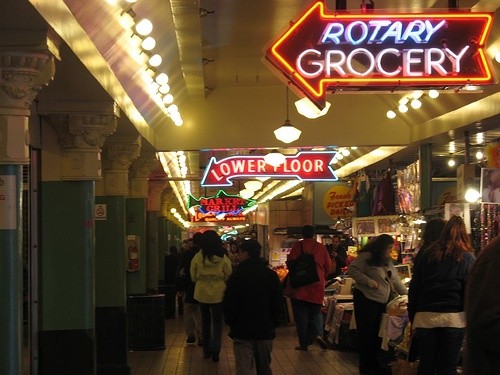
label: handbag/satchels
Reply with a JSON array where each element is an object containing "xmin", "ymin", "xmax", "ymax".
[{"xmin": 284, "ymin": 241, "xmax": 320, "ymax": 289}]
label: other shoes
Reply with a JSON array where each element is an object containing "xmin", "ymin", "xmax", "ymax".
[
  {"xmin": 212, "ymin": 356, "xmax": 219, "ymax": 362},
  {"xmin": 204, "ymin": 354, "xmax": 211, "ymax": 358},
  {"xmin": 186, "ymin": 336, "xmax": 196, "ymax": 345},
  {"xmin": 315, "ymin": 336, "xmax": 328, "ymax": 350},
  {"xmin": 295, "ymin": 346, "xmax": 308, "ymax": 351},
  {"xmin": 198, "ymin": 340, "xmax": 203, "ymax": 345}
]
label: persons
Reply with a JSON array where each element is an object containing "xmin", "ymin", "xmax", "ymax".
[
  {"xmin": 408, "ymin": 216, "xmax": 500, "ymax": 375},
  {"xmin": 282, "ymin": 226, "xmax": 347, "ymax": 351},
  {"xmin": 165, "ymin": 226, "xmax": 257, "ymax": 361},
  {"xmin": 223, "ymin": 240, "xmax": 282, "ymax": 375},
  {"xmin": 348, "ymin": 234, "xmax": 408, "ymax": 375}
]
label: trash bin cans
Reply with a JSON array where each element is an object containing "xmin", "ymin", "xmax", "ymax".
[
  {"xmin": 127, "ymin": 293, "xmax": 166, "ymax": 352},
  {"xmin": 148, "ymin": 285, "xmax": 175, "ymax": 319}
]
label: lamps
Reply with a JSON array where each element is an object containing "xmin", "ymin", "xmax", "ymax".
[
  {"xmin": 293, "ymin": 96, "xmax": 331, "ymax": 120},
  {"xmin": 273, "ymin": 86, "xmax": 302, "ymax": 144}
]
